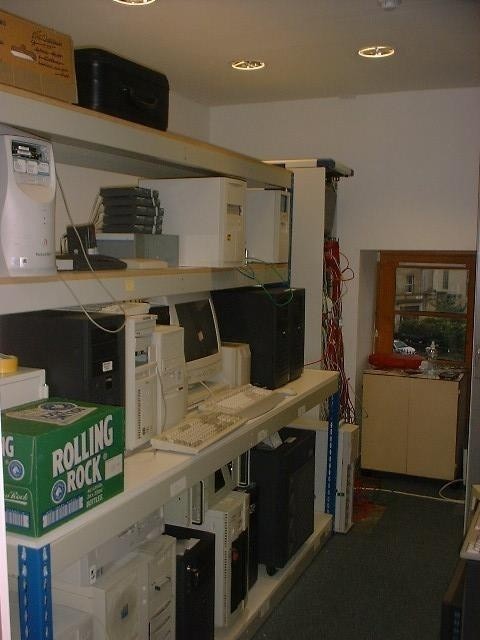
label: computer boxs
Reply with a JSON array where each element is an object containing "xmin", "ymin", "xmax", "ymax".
[
  {"xmin": 125, "ymin": 314, "xmax": 158, "ymax": 454},
  {"xmin": 246, "ymin": 190, "xmax": 290, "ymax": 263},
  {"xmin": 211, "ymin": 289, "xmax": 290, "ymax": 390},
  {"xmin": 0, "ymin": 135, "xmax": 57, "ymax": 278},
  {"xmin": 0, "ymin": 310, "xmax": 125, "ymax": 407},
  {"xmin": 243, "ymin": 286, "xmax": 305, "ymax": 381},
  {"xmin": 162, "ymin": 524, "xmax": 214, "ymax": 640},
  {"xmin": 138, "ymin": 177, "xmax": 246, "ymax": 269},
  {"xmin": 155, "ymin": 325, "xmax": 187, "ymax": 435}
]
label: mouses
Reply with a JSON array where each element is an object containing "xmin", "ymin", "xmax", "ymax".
[{"xmin": 272, "ymin": 388, "xmax": 297, "ymax": 396}]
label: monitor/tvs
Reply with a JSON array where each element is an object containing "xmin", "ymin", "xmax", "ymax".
[{"xmin": 142, "ymin": 290, "xmax": 227, "ymax": 406}]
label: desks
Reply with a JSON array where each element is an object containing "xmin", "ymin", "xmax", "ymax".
[{"xmin": 6, "ymin": 370, "xmax": 339, "ymax": 640}]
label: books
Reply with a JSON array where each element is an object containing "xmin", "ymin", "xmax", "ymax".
[{"xmin": 99, "ymin": 185, "xmax": 165, "ymax": 235}]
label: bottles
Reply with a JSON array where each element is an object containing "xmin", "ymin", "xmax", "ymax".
[{"xmin": 427, "ymin": 340, "xmax": 438, "ymax": 380}]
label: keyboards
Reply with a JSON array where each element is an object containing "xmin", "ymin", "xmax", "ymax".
[
  {"xmin": 199, "ymin": 384, "xmax": 285, "ymax": 420},
  {"xmin": 151, "ymin": 412, "xmax": 248, "ymax": 454}
]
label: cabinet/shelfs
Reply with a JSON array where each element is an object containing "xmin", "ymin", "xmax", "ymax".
[
  {"xmin": 359, "ymin": 368, "xmax": 467, "ymax": 481},
  {"xmin": 0, "ymin": 80, "xmax": 295, "ymax": 319}
]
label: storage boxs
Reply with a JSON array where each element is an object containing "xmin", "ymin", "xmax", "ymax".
[
  {"xmin": 1, "ymin": 10, "xmax": 80, "ymax": 105},
  {"xmin": 1, "ymin": 398, "xmax": 126, "ymax": 538}
]
